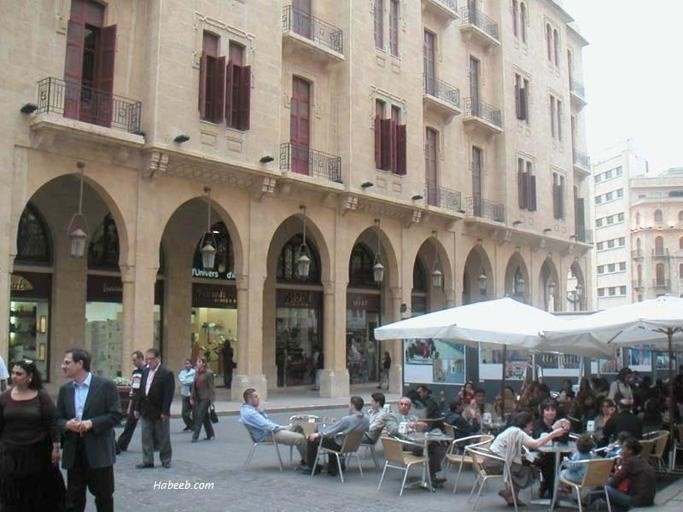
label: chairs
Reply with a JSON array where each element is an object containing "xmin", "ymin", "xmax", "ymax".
[{"xmin": 235, "ymin": 383, "xmax": 682, "ymax": 511}]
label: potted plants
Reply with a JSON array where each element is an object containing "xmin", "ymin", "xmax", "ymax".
[{"xmin": 113, "ymin": 377, "xmax": 131, "ymax": 414}]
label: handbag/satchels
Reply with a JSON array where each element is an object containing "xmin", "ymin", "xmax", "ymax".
[{"xmin": 210, "ymin": 409, "xmax": 218, "ymax": 423}]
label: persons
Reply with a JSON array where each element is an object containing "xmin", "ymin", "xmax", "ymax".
[
  {"xmin": 56, "ymin": 347, "xmax": 123, "ymax": 511},
  {"xmin": 190, "ymin": 356, "xmax": 216, "ymax": 443},
  {"xmin": 115, "ymin": 351, "xmax": 159, "ymax": 452},
  {"xmin": 0, "ymin": 359, "xmax": 56, "ymax": 512},
  {"xmin": 375, "ymin": 350, "xmax": 391, "ymax": 391},
  {"xmin": 0, "ymin": 356, "xmax": 10, "ymax": 393},
  {"xmin": 176, "ymin": 358, "xmax": 197, "ymax": 431},
  {"xmin": 132, "ymin": 348, "xmax": 175, "ymax": 469},
  {"xmin": 384, "ymin": 365, "xmax": 683, "ymax": 512},
  {"xmin": 239, "ymin": 388, "xmax": 324, "ymax": 469},
  {"xmin": 360, "ymin": 393, "xmax": 388, "ymax": 444},
  {"xmin": 297, "ymin": 396, "xmax": 370, "ymax": 475},
  {"xmin": 221, "ymin": 339, "xmax": 233, "ymax": 389}
]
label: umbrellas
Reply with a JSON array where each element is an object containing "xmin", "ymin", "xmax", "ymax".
[
  {"xmin": 538, "ymin": 293, "xmax": 683, "ymax": 440},
  {"xmin": 373, "ymin": 293, "xmax": 615, "ymax": 425}
]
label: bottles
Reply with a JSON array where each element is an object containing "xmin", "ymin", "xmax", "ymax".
[
  {"xmin": 608, "ymin": 434, "xmax": 615, "ymax": 445},
  {"xmin": 482, "ymin": 412, "xmax": 502, "ymax": 423}
]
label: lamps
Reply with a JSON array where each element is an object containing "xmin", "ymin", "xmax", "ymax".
[
  {"xmin": 198, "ymin": 186, "xmax": 218, "ymax": 269},
  {"xmin": 294, "ymin": 204, "xmax": 312, "ymax": 278},
  {"xmin": 371, "ymin": 217, "xmax": 583, "ymax": 298},
  {"xmin": 61, "ymin": 162, "xmax": 88, "ymax": 258}
]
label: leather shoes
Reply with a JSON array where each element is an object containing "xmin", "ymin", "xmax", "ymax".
[
  {"xmin": 162, "ymin": 462, "xmax": 170, "ymax": 467},
  {"xmin": 136, "ymin": 463, "xmax": 154, "ymax": 467}
]
